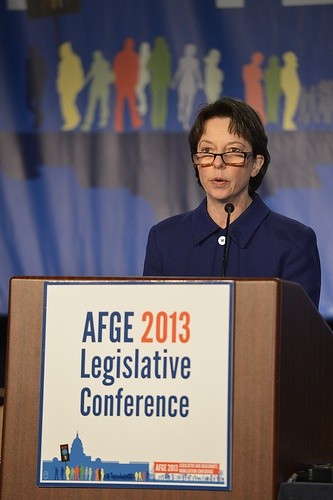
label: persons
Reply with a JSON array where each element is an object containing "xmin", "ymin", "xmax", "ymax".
[{"xmin": 136, "ymin": 95, "xmax": 323, "ymax": 319}]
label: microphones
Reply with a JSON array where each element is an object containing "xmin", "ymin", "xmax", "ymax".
[{"xmin": 220, "ymin": 202, "xmax": 234, "ymax": 277}]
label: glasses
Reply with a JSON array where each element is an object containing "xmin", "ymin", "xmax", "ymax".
[{"xmin": 192, "ymin": 150, "xmax": 254, "ymax": 167}]
